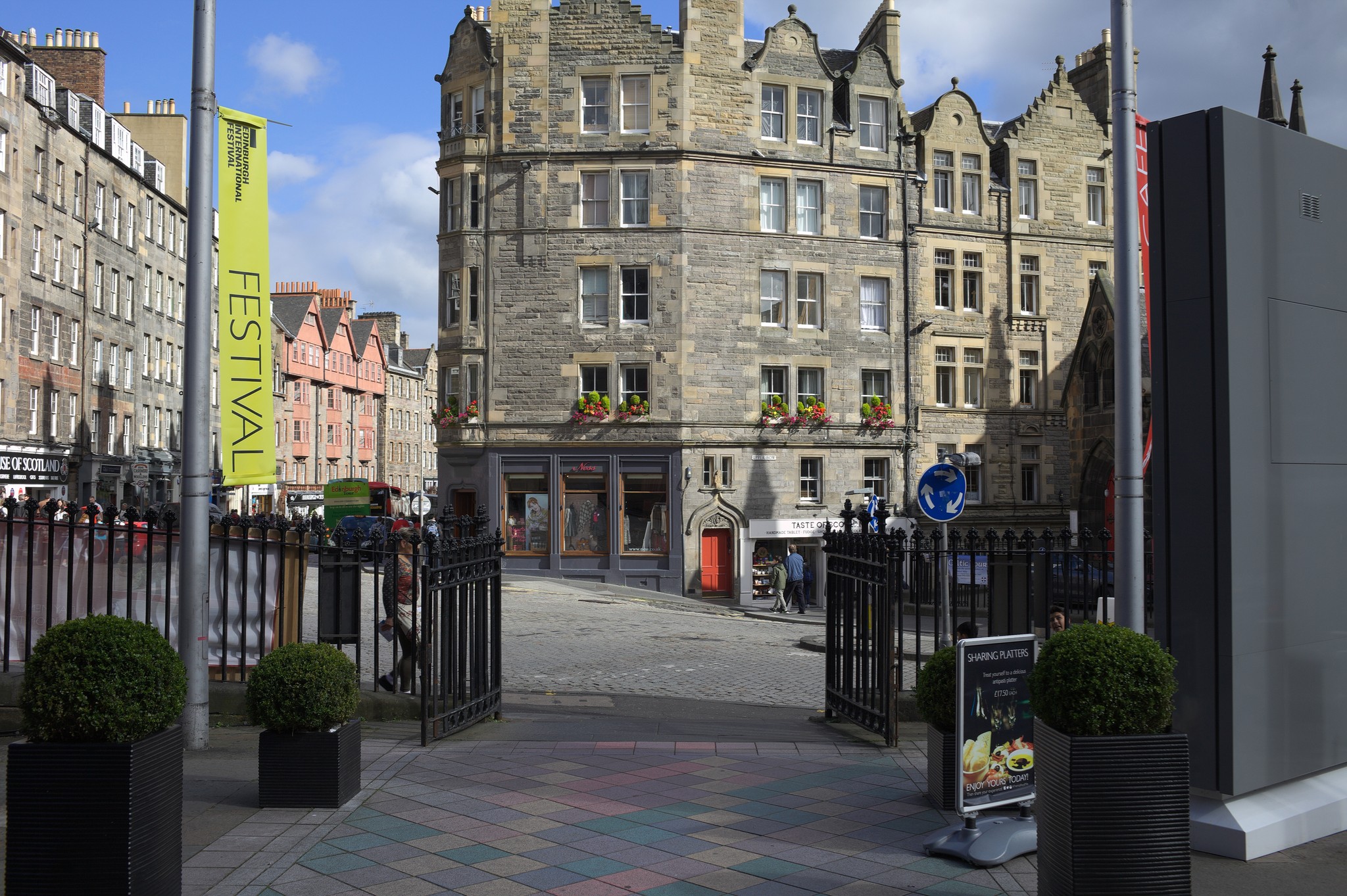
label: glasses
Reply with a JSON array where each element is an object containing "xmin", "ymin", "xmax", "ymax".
[{"xmin": 281, "ymin": 516, "xmax": 283, "ymax": 517}]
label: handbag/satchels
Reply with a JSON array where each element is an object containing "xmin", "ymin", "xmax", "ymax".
[
  {"xmin": 508, "ymin": 515, "xmax": 517, "ymax": 525},
  {"xmin": 396, "ymin": 589, "xmax": 435, "ymax": 646},
  {"xmin": 576, "ymin": 537, "xmax": 591, "ymax": 551}
]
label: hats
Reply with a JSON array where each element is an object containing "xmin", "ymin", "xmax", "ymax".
[{"xmin": 270, "ymin": 511, "xmax": 274, "ymax": 514}]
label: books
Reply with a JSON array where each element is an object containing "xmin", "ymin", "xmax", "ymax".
[{"xmin": 378, "ymin": 619, "xmax": 394, "ymax": 642}]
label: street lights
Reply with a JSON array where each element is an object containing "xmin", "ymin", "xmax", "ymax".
[
  {"xmin": 933, "ymin": 447, "xmax": 983, "ymax": 648},
  {"xmin": 845, "ymin": 487, "xmax": 876, "ymax": 535}
]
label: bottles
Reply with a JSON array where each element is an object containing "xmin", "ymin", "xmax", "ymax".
[{"xmin": 972, "ymin": 686, "xmax": 991, "ymax": 736}]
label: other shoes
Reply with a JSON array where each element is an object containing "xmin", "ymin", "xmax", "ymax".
[
  {"xmin": 793, "ymin": 600, "xmax": 798, "ymax": 605},
  {"xmin": 798, "ymin": 611, "xmax": 806, "ymax": 614},
  {"xmin": 806, "ymin": 604, "xmax": 810, "ymax": 608},
  {"xmin": 379, "ymin": 675, "xmax": 394, "ymax": 692},
  {"xmin": 776, "ymin": 607, "xmax": 781, "ymax": 612},
  {"xmin": 378, "ymin": 562, "xmax": 384, "ymax": 565}
]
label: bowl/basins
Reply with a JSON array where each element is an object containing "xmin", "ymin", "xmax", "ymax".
[{"xmin": 1006, "ymin": 749, "xmax": 1034, "ymax": 772}]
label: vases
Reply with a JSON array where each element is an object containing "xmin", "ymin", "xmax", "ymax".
[
  {"xmin": 468, "ymin": 416, "xmax": 478, "ymax": 423},
  {"xmin": 583, "ymin": 414, "xmax": 608, "ymax": 422},
  {"xmin": 863, "ymin": 418, "xmax": 892, "ymax": 426},
  {"xmin": 620, "ymin": 415, "xmax": 649, "ymax": 422},
  {"xmin": 799, "ymin": 418, "xmax": 817, "ymax": 424},
  {"xmin": 762, "ymin": 416, "xmax": 786, "ymax": 423},
  {"xmin": 439, "ymin": 418, "xmax": 444, "ymax": 424}
]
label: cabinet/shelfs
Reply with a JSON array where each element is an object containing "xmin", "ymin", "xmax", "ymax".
[{"xmin": 752, "ymin": 539, "xmax": 777, "ymax": 600}]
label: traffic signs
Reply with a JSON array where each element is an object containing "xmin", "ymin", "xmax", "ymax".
[{"xmin": 130, "ymin": 480, "xmax": 151, "ymax": 488}]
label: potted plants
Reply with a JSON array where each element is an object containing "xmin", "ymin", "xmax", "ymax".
[
  {"xmin": 245, "ymin": 641, "xmax": 363, "ymax": 807},
  {"xmin": 909, "ymin": 645, "xmax": 1022, "ymax": 813},
  {"xmin": 3, "ymin": 613, "xmax": 189, "ymax": 896},
  {"xmin": 448, "ymin": 396, "xmax": 459, "ymax": 423},
  {"xmin": 1027, "ymin": 621, "xmax": 1193, "ymax": 896},
  {"xmin": 971, "ymin": 397, "xmax": 978, "ymax": 404}
]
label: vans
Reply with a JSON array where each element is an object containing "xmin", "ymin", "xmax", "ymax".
[
  {"xmin": 326, "ymin": 514, "xmax": 395, "ymax": 560},
  {"xmin": 156, "ymin": 502, "xmax": 225, "ymax": 529}
]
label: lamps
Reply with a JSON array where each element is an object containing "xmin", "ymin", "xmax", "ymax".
[
  {"xmin": 428, "ymin": 186, "xmax": 440, "ymax": 195},
  {"xmin": 916, "ymin": 320, "xmax": 933, "ymax": 330},
  {"xmin": 88, "ymin": 222, "xmax": 99, "ymax": 230},
  {"xmin": 520, "ymin": 159, "xmax": 533, "ymax": 174}
]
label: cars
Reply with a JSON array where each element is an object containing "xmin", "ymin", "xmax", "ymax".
[{"xmin": 20, "ymin": 520, "xmax": 175, "ymax": 562}]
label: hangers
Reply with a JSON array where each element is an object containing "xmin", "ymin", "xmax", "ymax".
[
  {"xmin": 586, "ymin": 496, "xmax": 590, "ymax": 503},
  {"xmin": 569, "ymin": 502, "xmax": 573, "ymax": 508}
]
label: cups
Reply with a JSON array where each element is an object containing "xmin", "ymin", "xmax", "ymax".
[
  {"xmin": 991, "ymin": 701, "xmax": 1004, "ymax": 747},
  {"xmin": 962, "ymin": 755, "xmax": 990, "ymax": 789},
  {"xmin": 1006, "ymin": 699, "xmax": 1021, "ymax": 740}
]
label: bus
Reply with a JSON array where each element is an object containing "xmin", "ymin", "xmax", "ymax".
[{"xmin": 368, "ymin": 481, "xmax": 439, "ymax": 519}]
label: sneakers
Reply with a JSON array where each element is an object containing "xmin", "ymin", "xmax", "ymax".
[
  {"xmin": 780, "ymin": 610, "xmax": 789, "ymax": 614},
  {"xmin": 768, "ymin": 608, "xmax": 776, "ymax": 613}
]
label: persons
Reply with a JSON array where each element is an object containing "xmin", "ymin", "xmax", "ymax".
[
  {"xmin": 0, "ymin": 520, "xmax": 128, "ymax": 567},
  {"xmin": 769, "ymin": 555, "xmax": 789, "ymax": 614},
  {"xmin": 422, "ymin": 513, "xmax": 442, "ymax": 568},
  {"xmin": 1050, "ymin": 604, "xmax": 1074, "ymax": 633},
  {"xmin": 0, "ymin": 486, "xmax": 159, "ymax": 528},
  {"xmin": 369, "ymin": 516, "xmax": 388, "ymax": 566},
  {"xmin": 229, "ymin": 509, "xmax": 323, "ymax": 538},
  {"xmin": 414, "ymin": 516, "xmax": 425, "ymax": 529},
  {"xmin": 377, "ymin": 527, "xmax": 423, "ymax": 693},
  {"xmin": 526, "ymin": 497, "xmax": 548, "ymax": 533},
  {"xmin": 957, "ymin": 621, "xmax": 979, "ymax": 641},
  {"xmin": 776, "ymin": 544, "xmax": 806, "ymax": 614},
  {"xmin": 793, "ymin": 557, "xmax": 811, "ymax": 608},
  {"xmin": 391, "ymin": 512, "xmax": 410, "ymax": 533}
]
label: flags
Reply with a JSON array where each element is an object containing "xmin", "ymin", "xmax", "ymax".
[{"xmin": 214, "ymin": 105, "xmax": 278, "ymax": 487}]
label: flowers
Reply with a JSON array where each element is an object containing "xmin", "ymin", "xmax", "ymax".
[
  {"xmin": 795, "ymin": 395, "xmax": 833, "ymax": 427},
  {"xmin": 861, "ymin": 392, "xmax": 895, "ymax": 429},
  {"xmin": 429, "ymin": 405, "xmax": 456, "ymax": 429},
  {"xmin": 459, "ymin": 400, "xmax": 479, "ymax": 420},
  {"xmin": 570, "ymin": 389, "xmax": 610, "ymax": 424},
  {"xmin": 615, "ymin": 395, "xmax": 651, "ymax": 421},
  {"xmin": 760, "ymin": 395, "xmax": 796, "ymax": 427}
]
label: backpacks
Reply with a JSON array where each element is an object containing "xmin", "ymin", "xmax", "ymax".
[
  {"xmin": 305, "ymin": 517, "xmax": 310, "ymax": 527},
  {"xmin": 232, "ymin": 514, "xmax": 238, "ymax": 524},
  {"xmin": 425, "ymin": 522, "xmax": 439, "ymax": 542},
  {"xmin": 374, "ymin": 523, "xmax": 384, "ymax": 531},
  {"xmin": 803, "ymin": 567, "xmax": 813, "ymax": 583}
]
